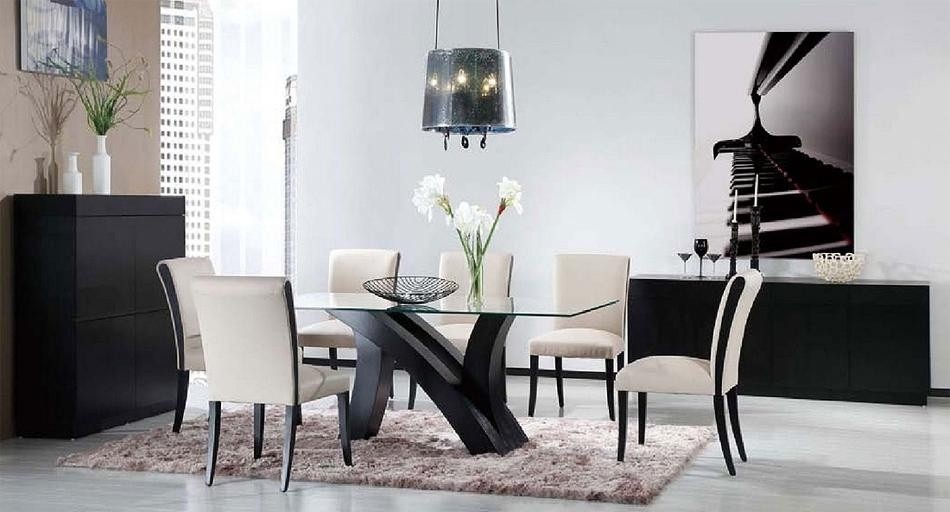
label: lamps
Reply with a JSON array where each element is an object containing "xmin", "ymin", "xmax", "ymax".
[{"xmin": 420, "ymin": 0, "xmax": 515, "ymax": 154}]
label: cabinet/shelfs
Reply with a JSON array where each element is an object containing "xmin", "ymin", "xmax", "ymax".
[
  {"xmin": 14, "ymin": 193, "xmax": 190, "ymax": 438},
  {"xmin": 629, "ymin": 271, "xmax": 935, "ymax": 405}
]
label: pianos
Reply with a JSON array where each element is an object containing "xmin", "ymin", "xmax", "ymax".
[{"xmin": 694, "ymin": 31, "xmax": 855, "ymax": 259}]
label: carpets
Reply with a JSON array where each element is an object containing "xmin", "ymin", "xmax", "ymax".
[{"xmin": 54, "ymin": 404, "xmax": 720, "ymax": 506}]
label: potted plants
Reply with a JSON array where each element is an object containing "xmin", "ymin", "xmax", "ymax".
[
  {"xmin": 42, "ymin": 38, "xmax": 153, "ymax": 196},
  {"xmin": 20, "ymin": 59, "xmax": 77, "ymax": 198}
]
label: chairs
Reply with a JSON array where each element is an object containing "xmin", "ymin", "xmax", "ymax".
[
  {"xmin": 525, "ymin": 252, "xmax": 633, "ymax": 418},
  {"xmin": 613, "ymin": 268, "xmax": 764, "ymax": 476},
  {"xmin": 190, "ymin": 274, "xmax": 354, "ymax": 490},
  {"xmin": 407, "ymin": 249, "xmax": 517, "ymax": 409},
  {"xmin": 298, "ymin": 246, "xmax": 400, "ymax": 398},
  {"xmin": 158, "ymin": 253, "xmax": 309, "ymax": 433}
]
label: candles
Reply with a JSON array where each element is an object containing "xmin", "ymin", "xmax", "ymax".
[
  {"xmin": 732, "ymin": 186, "xmax": 740, "ymax": 223},
  {"xmin": 751, "ymin": 172, "xmax": 761, "ymax": 206}
]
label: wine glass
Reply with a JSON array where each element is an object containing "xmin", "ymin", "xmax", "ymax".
[
  {"xmin": 694, "ymin": 238, "xmax": 708, "ymax": 279},
  {"xmin": 678, "ymin": 252, "xmax": 692, "ymax": 278},
  {"xmin": 707, "ymin": 252, "xmax": 722, "ymax": 278}
]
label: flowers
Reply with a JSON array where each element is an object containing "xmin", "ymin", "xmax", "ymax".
[{"xmin": 414, "ymin": 176, "xmax": 527, "ymax": 246}]
